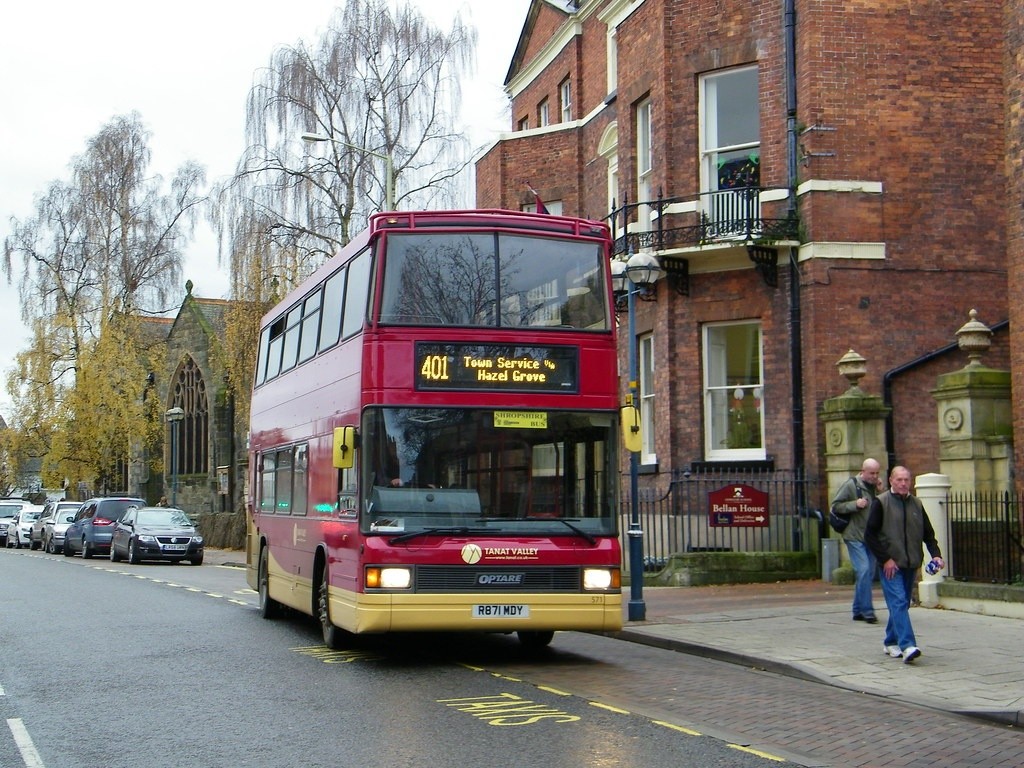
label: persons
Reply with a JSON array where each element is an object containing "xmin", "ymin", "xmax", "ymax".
[
  {"xmin": 155, "ymin": 496, "xmax": 169, "ymax": 507},
  {"xmin": 864, "ymin": 466, "xmax": 945, "ymax": 663},
  {"xmin": 372, "ymin": 442, "xmax": 436, "ymax": 489},
  {"xmin": 832, "ymin": 458, "xmax": 885, "ymax": 623}
]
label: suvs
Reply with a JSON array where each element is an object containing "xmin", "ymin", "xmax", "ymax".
[{"xmin": 62, "ymin": 497, "xmax": 148, "ymax": 557}]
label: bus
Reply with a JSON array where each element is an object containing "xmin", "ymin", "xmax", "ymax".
[{"xmin": 246, "ymin": 204, "xmax": 646, "ymax": 650}]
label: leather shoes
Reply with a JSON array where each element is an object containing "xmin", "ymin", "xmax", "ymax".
[{"xmin": 852, "ymin": 613, "xmax": 877, "ymax": 623}]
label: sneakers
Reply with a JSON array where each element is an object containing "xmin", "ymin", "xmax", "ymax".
[{"xmin": 882, "ymin": 640, "xmax": 921, "ymax": 664}]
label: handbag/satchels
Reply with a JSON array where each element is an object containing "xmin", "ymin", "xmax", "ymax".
[{"xmin": 828, "ymin": 476, "xmax": 863, "ymax": 534}]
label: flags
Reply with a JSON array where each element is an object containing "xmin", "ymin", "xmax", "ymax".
[{"xmin": 528, "ymin": 184, "xmax": 549, "ymax": 214}]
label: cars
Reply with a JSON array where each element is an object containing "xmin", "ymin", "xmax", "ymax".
[
  {"xmin": 110, "ymin": 502, "xmax": 205, "ymax": 567},
  {"xmin": 0, "ymin": 498, "xmax": 86, "ymax": 554}
]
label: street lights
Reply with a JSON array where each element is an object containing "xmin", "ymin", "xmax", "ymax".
[
  {"xmin": 302, "ymin": 132, "xmax": 393, "ymax": 211},
  {"xmin": 611, "ymin": 255, "xmax": 660, "ymax": 620},
  {"xmin": 166, "ymin": 407, "xmax": 185, "ymax": 508}
]
